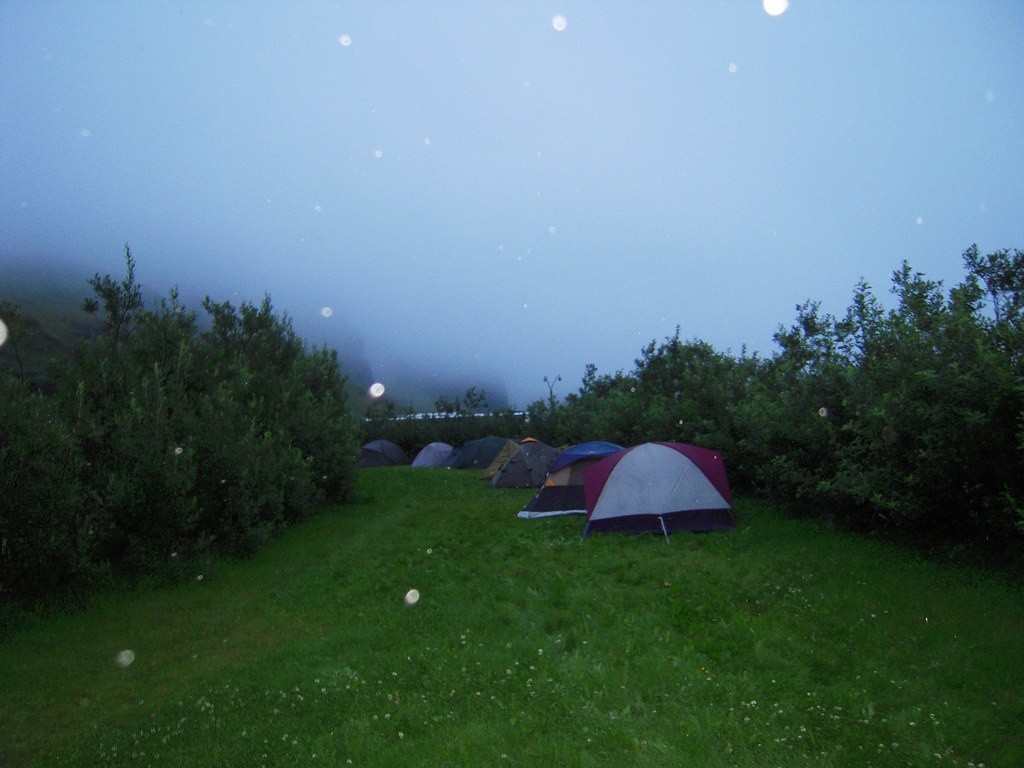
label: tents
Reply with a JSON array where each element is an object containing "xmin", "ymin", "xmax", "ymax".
[
  {"xmin": 583, "ymin": 442, "xmax": 735, "ymax": 536},
  {"xmin": 442, "ymin": 436, "xmax": 510, "ymax": 469},
  {"xmin": 412, "ymin": 442, "xmax": 453, "ymax": 467},
  {"xmin": 492, "ymin": 439, "xmax": 560, "ymax": 488},
  {"xmin": 478, "ymin": 439, "xmax": 521, "ymax": 480},
  {"xmin": 352, "ymin": 440, "xmax": 413, "ymax": 467},
  {"xmin": 518, "ymin": 442, "xmax": 626, "ymax": 518}
]
